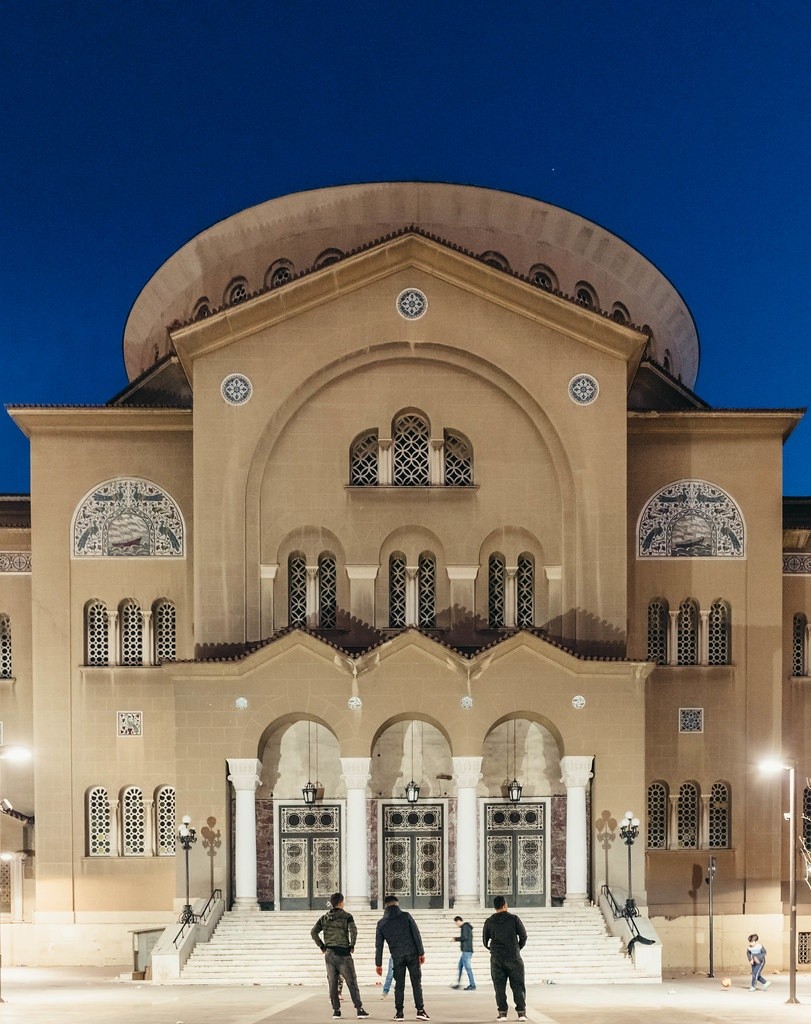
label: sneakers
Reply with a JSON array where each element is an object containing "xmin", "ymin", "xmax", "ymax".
[
  {"xmin": 415, "ymin": 1009, "xmax": 430, "ymax": 1021},
  {"xmin": 496, "ymin": 1014, "xmax": 507, "ymax": 1022},
  {"xmin": 332, "ymin": 1010, "xmax": 342, "ymax": 1019},
  {"xmin": 517, "ymin": 1012, "xmax": 528, "ymax": 1021},
  {"xmin": 357, "ymin": 1008, "xmax": 370, "ymax": 1019},
  {"xmin": 392, "ymin": 1012, "xmax": 405, "ymax": 1021}
]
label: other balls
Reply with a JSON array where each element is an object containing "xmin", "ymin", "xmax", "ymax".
[{"xmin": 721, "ymin": 977, "xmax": 731, "ymax": 987}]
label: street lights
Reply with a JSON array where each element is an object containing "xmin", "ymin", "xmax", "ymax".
[
  {"xmin": 758, "ymin": 756, "xmax": 799, "ymax": 1005},
  {"xmin": 178, "ymin": 813, "xmax": 198, "ymax": 923},
  {"xmin": 0, "ymin": 740, "xmax": 33, "ymax": 1005},
  {"xmin": 617, "ymin": 810, "xmax": 641, "ymax": 917}
]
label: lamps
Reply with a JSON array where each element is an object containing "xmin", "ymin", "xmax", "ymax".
[
  {"xmin": 404, "ymin": 720, "xmax": 420, "ymax": 811},
  {"xmin": 509, "ymin": 719, "xmax": 523, "ymax": 808},
  {"xmin": 302, "ymin": 721, "xmax": 316, "ymax": 810}
]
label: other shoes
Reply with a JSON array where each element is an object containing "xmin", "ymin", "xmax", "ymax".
[
  {"xmin": 337, "ymin": 992, "xmax": 344, "ymax": 1002},
  {"xmin": 762, "ymin": 982, "xmax": 771, "ymax": 990},
  {"xmin": 380, "ymin": 993, "xmax": 387, "ymax": 1000},
  {"xmin": 451, "ymin": 985, "xmax": 460, "ymax": 990},
  {"xmin": 464, "ymin": 983, "xmax": 476, "ymax": 990},
  {"xmin": 749, "ymin": 987, "xmax": 756, "ymax": 992},
  {"xmin": 329, "ymin": 998, "xmax": 332, "ymax": 1005}
]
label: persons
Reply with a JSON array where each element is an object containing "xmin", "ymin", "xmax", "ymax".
[
  {"xmin": 483, "ymin": 895, "xmax": 527, "ymax": 1022},
  {"xmin": 381, "ymin": 956, "xmax": 394, "ymax": 1000},
  {"xmin": 374, "ymin": 896, "xmax": 432, "ymax": 1021},
  {"xmin": 311, "ymin": 892, "xmax": 371, "ymax": 1019},
  {"xmin": 451, "ymin": 916, "xmax": 476, "ymax": 990},
  {"xmin": 746, "ymin": 934, "xmax": 772, "ymax": 992},
  {"xmin": 327, "ymin": 975, "xmax": 344, "ymax": 1003}
]
